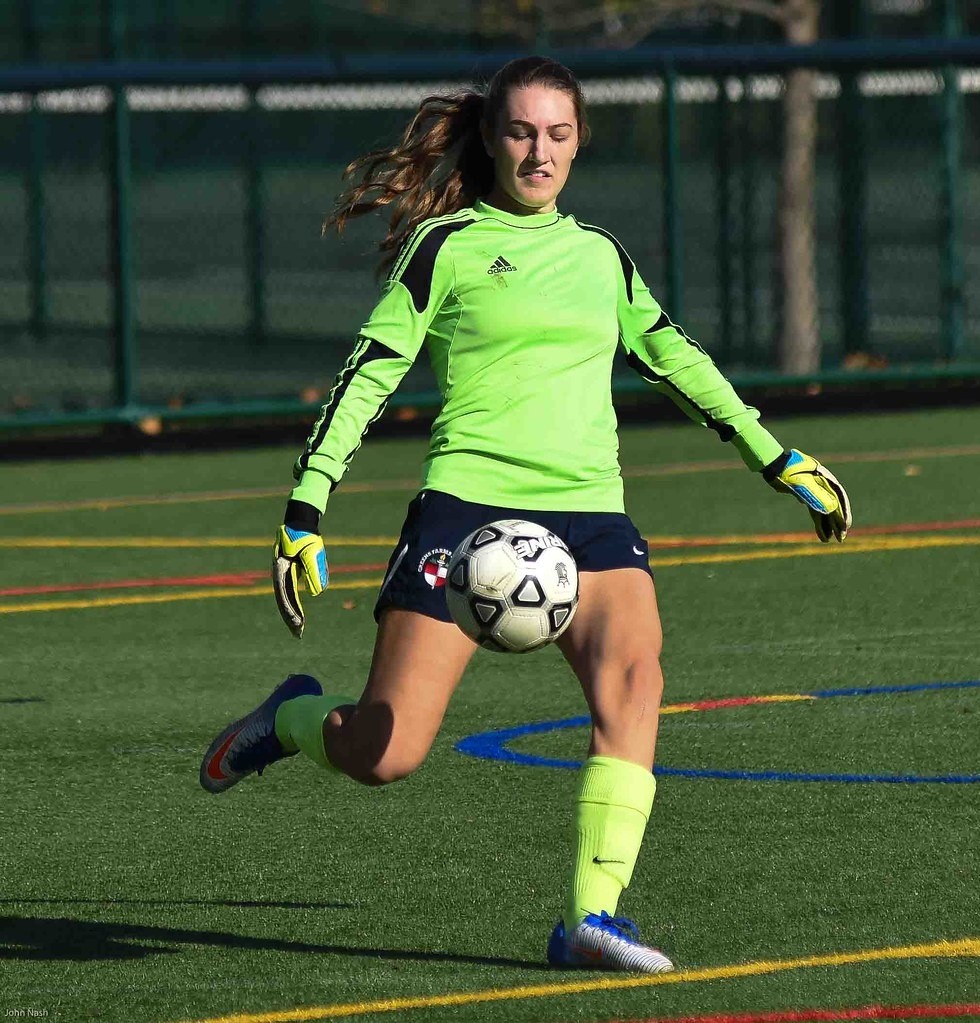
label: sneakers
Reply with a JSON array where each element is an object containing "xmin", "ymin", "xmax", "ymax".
[
  {"xmin": 200, "ymin": 675, "xmax": 320, "ymax": 795},
  {"xmin": 546, "ymin": 912, "xmax": 677, "ymax": 973}
]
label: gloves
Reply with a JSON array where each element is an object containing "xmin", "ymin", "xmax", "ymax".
[
  {"xmin": 272, "ymin": 499, "xmax": 327, "ymax": 639},
  {"xmin": 761, "ymin": 448, "xmax": 853, "ymax": 544}
]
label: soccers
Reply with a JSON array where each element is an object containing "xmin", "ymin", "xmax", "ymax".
[{"xmin": 444, "ymin": 518, "xmax": 581, "ymax": 656}]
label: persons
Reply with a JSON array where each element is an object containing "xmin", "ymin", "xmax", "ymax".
[{"xmin": 199, "ymin": 56, "xmax": 853, "ymax": 974}]
